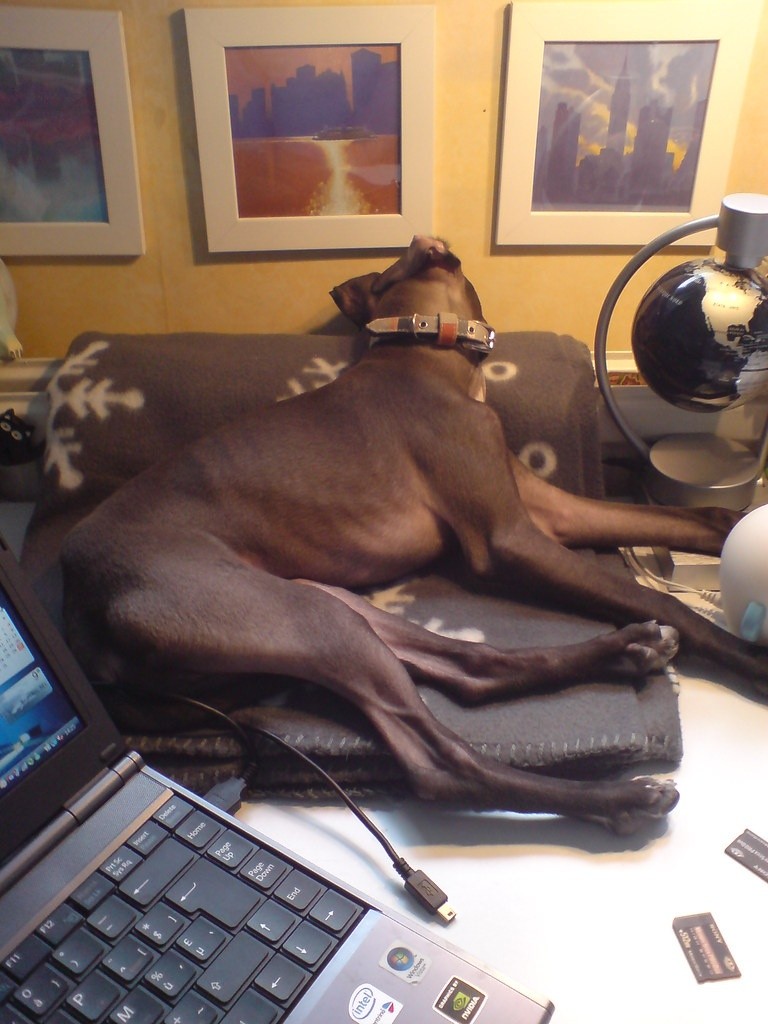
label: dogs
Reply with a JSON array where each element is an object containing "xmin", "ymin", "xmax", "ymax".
[{"xmin": 62, "ymin": 234, "xmax": 768, "ymax": 835}]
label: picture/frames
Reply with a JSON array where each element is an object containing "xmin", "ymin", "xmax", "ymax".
[
  {"xmin": 0, "ymin": 5, "xmax": 146, "ymax": 257},
  {"xmin": 184, "ymin": 2, "xmax": 438, "ymax": 254},
  {"xmin": 494, "ymin": 1, "xmax": 762, "ymax": 245}
]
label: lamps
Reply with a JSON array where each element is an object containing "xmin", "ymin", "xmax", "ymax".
[{"xmin": 594, "ymin": 193, "xmax": 768, "ymax": 643}]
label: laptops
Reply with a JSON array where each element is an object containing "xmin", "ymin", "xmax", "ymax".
[{"xmin": 1, "ymin": 529, "xmax": 559, "ymax": 1024}]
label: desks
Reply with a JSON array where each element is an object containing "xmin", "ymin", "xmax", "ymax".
[{"xmin": 0, "ymin": 497, "xmax": 768, "ymax": 1024}]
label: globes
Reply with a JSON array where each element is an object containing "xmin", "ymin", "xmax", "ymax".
[{"xmin": 594, "ymin": 193, "xmax": 768, "ymax": 513}]
